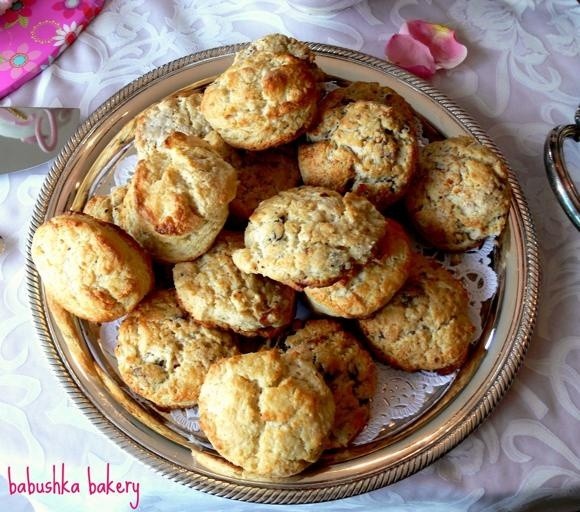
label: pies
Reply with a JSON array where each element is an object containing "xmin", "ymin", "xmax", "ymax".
[{"xmin": 29, "ymin": 32, "xmax": 511, "ymax": 477}]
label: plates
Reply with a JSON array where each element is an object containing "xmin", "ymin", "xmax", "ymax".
[{"xmin": 21, "ymin": 35, "xmax": 543, "ymax": 506}]
